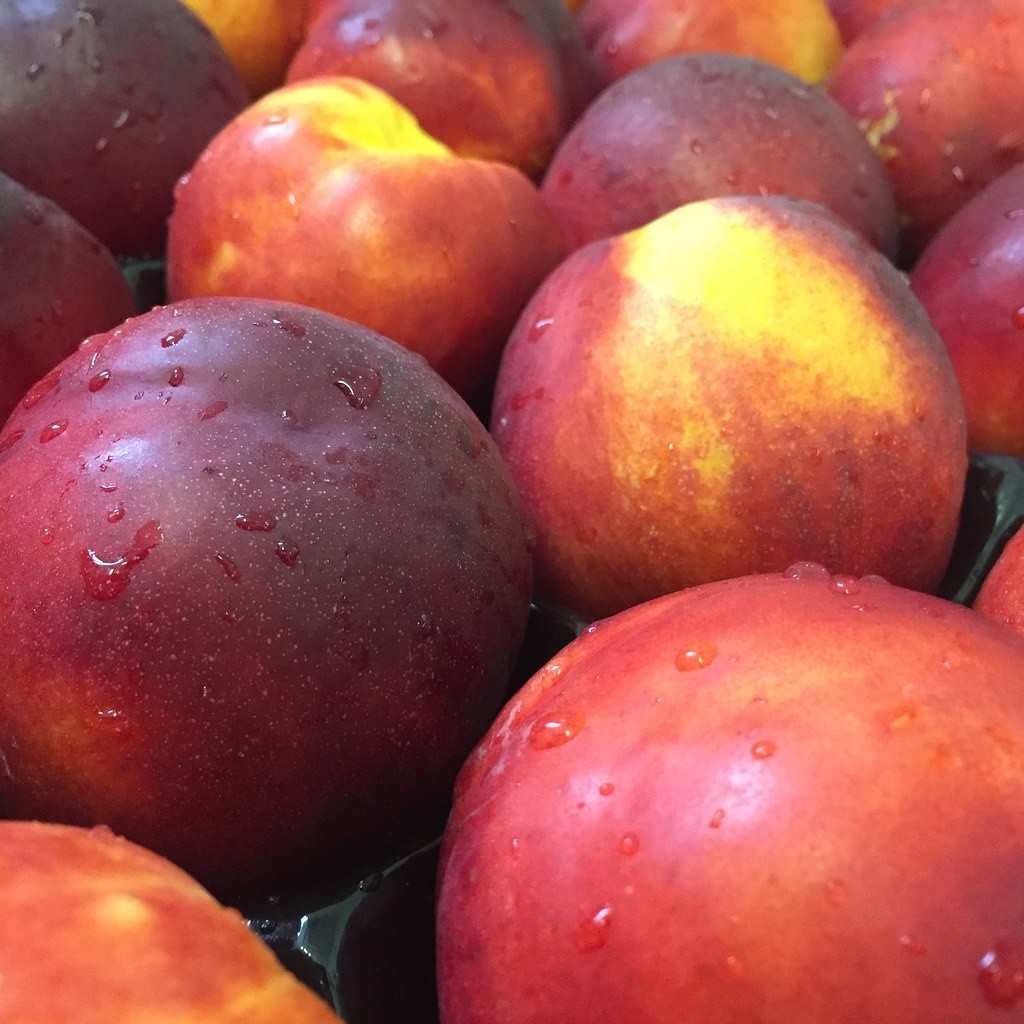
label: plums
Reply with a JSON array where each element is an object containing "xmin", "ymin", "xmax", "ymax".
[{"xmin": 0, "ymin": 0, "xmax": 1024, "ymax": 1024}]
